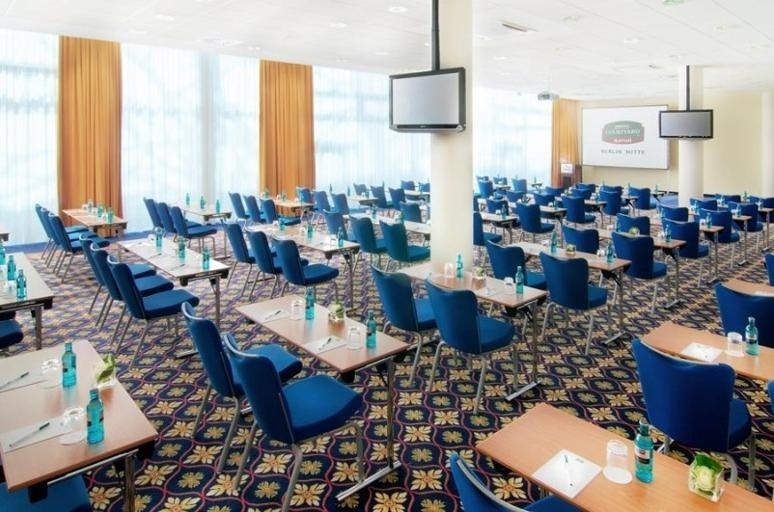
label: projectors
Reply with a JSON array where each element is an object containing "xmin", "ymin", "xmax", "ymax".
[{"xmin": 537, "ymin": 91, "xmax": 560, "ymax": 101}]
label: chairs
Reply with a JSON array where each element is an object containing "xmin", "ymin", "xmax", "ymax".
[{"xmin": 0, "ymin": 175, "xmax": 774, "ymax": 512}]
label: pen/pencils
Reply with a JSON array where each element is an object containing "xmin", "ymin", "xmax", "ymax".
[
  {"xmin": 0, "ymin": 372, "xmax": 29, "ymax": 390},
  {"xmin": 564, "ymin": 456, "xmax": 573, "ymax": 486},
  {"xmin": 270, "ymin": 310, "xmax": 281, "ymax": 318},
  {"xmin": 149, "ymin": 253, "xmax": 162, "ymax": 260},
  {"xmin": 321, "ymin": 338, "xmax": 332, "ymax": 349},
  {"xmin": 9, "ymin": 422, "xmax": 50, "ymax": 447},
  {"xmin": 172, "ymin": 264, "xmax": 185, "ymax": 270}
]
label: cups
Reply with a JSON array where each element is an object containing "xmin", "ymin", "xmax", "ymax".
[
  {"xmin": 292, "ymin": 300, "xmax": 305, "ymax": 322},
  {"xmin": 724, "ymin": 330, "xmax": 745, "ymax": 358},
  {"xmin": 57, "ymin": 406, "xmax": 85, "ymax": 444},
  {"xmin": 600, "ymin": 440, "xmax": 634, "ymax": 485},
  {"xmin": 40, "ymin": 355, "xmax": 63, "ymax": 389},
  {"xmin": 346, "ymin": 328, "xmax": 362, "ymax": 351}
]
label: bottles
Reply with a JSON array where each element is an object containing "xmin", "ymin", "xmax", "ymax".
[
  {"xmin": 304, "ymin": 289, "xmax": 314, "ymax": 321},
  {"xmin": 634, "ymin": 418, "xmax": 654, "ymax": 484},
  {"xmin": 364, "ymin": 311, "xmax": 377, "ymax": 348},
  {"xmin": 743, "ymin": 316, "xmax": 760, "ymax": 356},
  {"xmin": 83, "ymin": 388, "xmax": 106, "ymax": 449},
  {"xmin": 597, "ymin": 178, "xmax": 658, "ymax": 193},
  {"xmin": 275, "ymin": 215, "xmax": 346, "ymax": 244},
  {"xmin": 481, "ymin": 170, "xmax": 539, "ymax": 185},
  {"xmin": 453, "ymin": 194, "xmax": 765, "ymax": 296},
  {"xmin": 0, "ymin": 241, "xmax": 30, "ymax": 299},
  {"xmin": 60, "ymin": 340, "xmax": 84, "ymax": 391},
  {"xmin": 183, "ymin": 191, "xmax": 223, "ymax": 212},
  {"xmin": 149, "ymin": 225, "xmax": 214, "ymax": 270},
  {"xmin": 85, "ymin": 199, "xmax": 115, "ymax": 224},
  {"xmin": 322, "ymin": 179, "xmax": 427, "ymax": 227}
]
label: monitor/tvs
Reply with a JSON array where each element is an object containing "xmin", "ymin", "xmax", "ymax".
[
  {"xmin": 388, "ymin": 66, "xmax": 466, "ymax": 133},
  {"xmin": 658, "ymin": 110, "xmax": 713, "ymax": 139}
]
